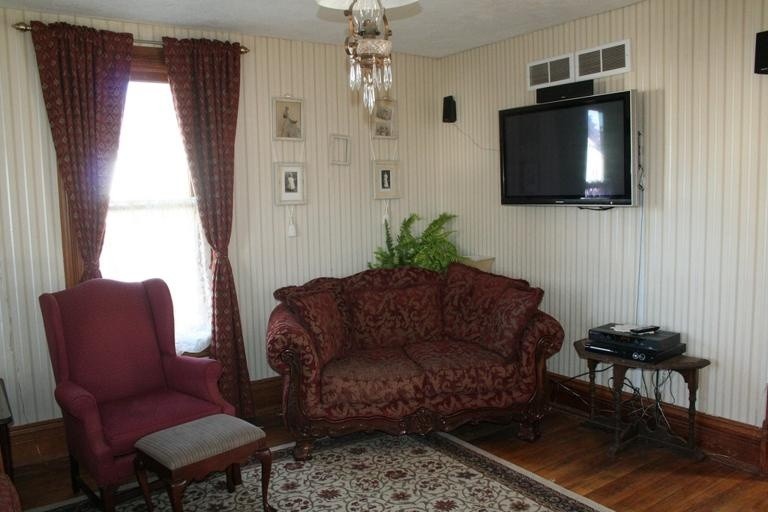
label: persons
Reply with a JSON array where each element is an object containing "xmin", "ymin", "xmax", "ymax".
[
  {"xmin": 285, "ymin": 172, "xmax": 296, "ymax": 192},
  {"xmin": 278, "ymin": 104, "xmax": 300, "ymax": 139}
]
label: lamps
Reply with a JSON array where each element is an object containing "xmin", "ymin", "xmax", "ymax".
[{"xmin": 343, "ymin": 0, "xmax": 393, "ymax": 113}]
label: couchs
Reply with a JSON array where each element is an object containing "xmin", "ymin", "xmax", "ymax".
[{"xmin": 265, "ymin": 264, "xmax": 564, "ymax": 461}]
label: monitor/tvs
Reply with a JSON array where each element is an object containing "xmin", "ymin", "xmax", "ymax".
[{"xmin": 497, "ymin": 86, "xmax": 639, "ymax": 208}]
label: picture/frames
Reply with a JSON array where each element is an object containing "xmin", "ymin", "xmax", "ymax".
[
  {"xmin": 329, "ymin": 134, "xmax": 350, "ymax": 165},
  {"xmin": 271, "ymin": 97, "xmax": 304, "ymax": 141},
  {"xmin": 373, "ymin": 159, "xmax": 402, "ymax": 199},
  {"xmin": 371, "ymin": 98, "xmax": 399, "ymax": 140},
  {"xmin": 273, "ymin": 162, "xmax": 308, "ymax": 206}
]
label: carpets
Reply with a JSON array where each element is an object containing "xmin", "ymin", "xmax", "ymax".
[{"xmin": 28, "ymin": 425, "xmax": 619, "ymax": 512}]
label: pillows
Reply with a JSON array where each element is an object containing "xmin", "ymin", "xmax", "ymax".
[{"xmin": 273, "ymin": 262, "xmax": 544, "ymax": 357}]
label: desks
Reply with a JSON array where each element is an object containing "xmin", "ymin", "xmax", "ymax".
[
  {"xmin": 574, "ymin": 338, "xmax": 711, "ymax": 460},
  {"xmin": 0, "ymin": 378, "xmax": 22, "ymax": 477}
]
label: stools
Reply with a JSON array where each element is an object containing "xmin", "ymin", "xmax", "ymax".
[{"xmin": 132, "ymin": 414, "xmax": 278, "ymax": 512}]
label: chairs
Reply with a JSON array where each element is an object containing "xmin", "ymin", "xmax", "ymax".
[
  {"xmin": 0, "ymin": 453, "xmax": 22, "ymax": 512},
  {"xmin": 39, "ymin": 278, "xmax": 243, "ymax": 512}
]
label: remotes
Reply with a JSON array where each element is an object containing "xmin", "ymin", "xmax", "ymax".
[{"xmin": 630, "ymin": 324, "xmax": 660, "ymax": 333}]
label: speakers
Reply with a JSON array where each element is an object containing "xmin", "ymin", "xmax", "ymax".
[
  {"xmin": 442, "ymin": 96, "xmax": 457, "ymax": 123},
  {"xmin": 754, "ymin": 30, "xmax": 768, "ymax": 75},
  {"xmin": 534, "ymin": 78, "xmax": 596, "ymax": 104}
]
